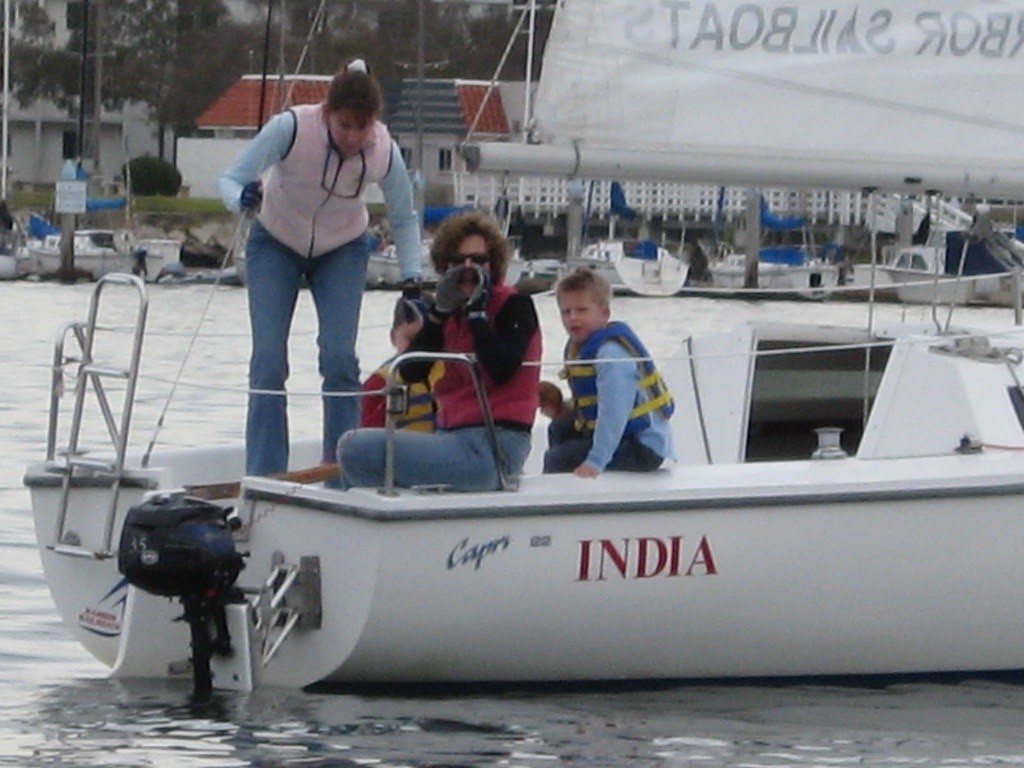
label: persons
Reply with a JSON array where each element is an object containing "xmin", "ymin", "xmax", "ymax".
[
  {"xmin": 360, "ymin": 292, "xmax": 446, "ymax": 434},
  {"xmin": 218, "ymin": 57, "xmax": 420, "ymax": 493},
  {"xmin": 544, "ymin": 271, "xmax": 675, "ymax": 479},
  {"xmin": 335, "ymin": 211, "xmax": 542, "ymax": 495}
]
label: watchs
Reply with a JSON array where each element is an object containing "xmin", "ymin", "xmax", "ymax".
[{"xmin": 402, "ymin": 277, "xmax": 418, "ymax": 286}]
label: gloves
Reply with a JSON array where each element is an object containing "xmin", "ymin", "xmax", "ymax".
[
  {"xmin": 433, "ymin": 264, "xmax": 464, "ymax": 313},
  {"xmin": 240, "ymin": 180, "xmax": 264, "ymax": 212},
  {"xmin": 466, "ymin": 269, "xmax": 490, "ymax": 317},
  {"xmin": 394, "ymin": 286, "xmax": 427, "ymax": 331}
]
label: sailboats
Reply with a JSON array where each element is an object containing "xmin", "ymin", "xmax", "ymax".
[{"xmin": 0, "ymin": 0, "xmax": 1024, "ymax": 696}]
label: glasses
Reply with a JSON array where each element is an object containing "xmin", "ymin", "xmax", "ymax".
[{"xmin": 448, "ymin": 252, "xmax": 494, "ymax": 266}]
